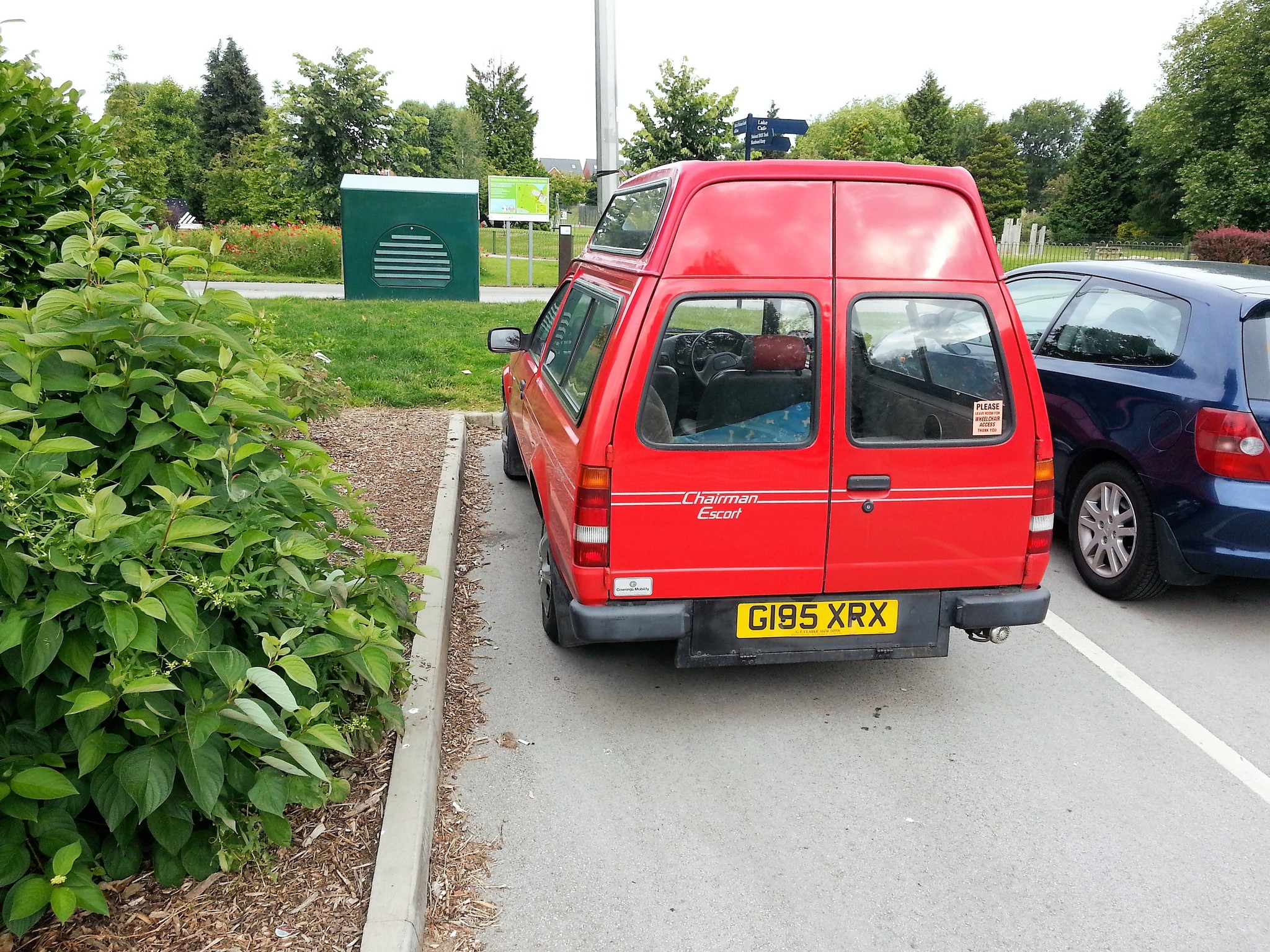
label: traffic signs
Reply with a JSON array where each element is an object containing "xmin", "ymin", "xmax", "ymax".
[
  {"xmin": 750, "ymin": 127, "xmax": 775, "ymax": 147},
  {"xmin": 733, "ymin": 118, "xmax": 747, "ymax": 136},
  {"xmin": 751, "ymin": 117, "xmax": 809, "ymax": 136},
  {"xmin": 751, "ymin": 136, "xmax": 791, "ymax": 151}
]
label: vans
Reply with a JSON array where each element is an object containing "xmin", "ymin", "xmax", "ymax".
[{"xmin": 487, "ymin": 160, "xmax": 1054, "ymax": 668}]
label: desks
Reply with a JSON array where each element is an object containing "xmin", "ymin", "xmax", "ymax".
[{"xmin": 1090, "ymin": 247, "xmax": 1122, "ymax": 259}]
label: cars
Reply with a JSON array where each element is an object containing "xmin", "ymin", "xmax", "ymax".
[{"xmin": 867, "ymin": 258, "xmax": 1270, "ymax": 603}]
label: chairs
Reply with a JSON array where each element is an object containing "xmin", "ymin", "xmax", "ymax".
[
  {"xmin": 1094, "ymin": 309, "xmax": 1167, "ymax": 362},
  {"xmin": 679, "ymin": 333, "xmax": 812, "ymax": 434},
  {"xmin": 644, "ymin": 354, "xmax": 679, "ymax": 436}
]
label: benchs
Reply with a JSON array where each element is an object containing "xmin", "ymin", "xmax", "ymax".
[
  {"xmin": 1098, "ymin": 252, "xmax": 1128, "ymax": 258},
  {"xmin": 1081, "ymin": 251, "xmax": 1090, "ymax": 255}
]
